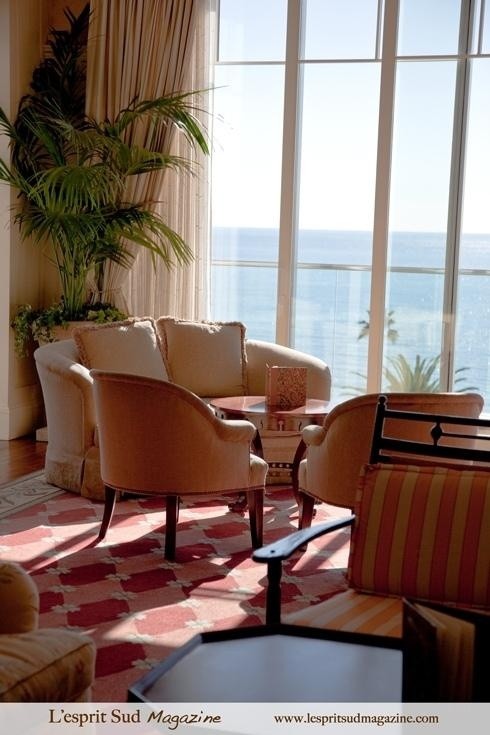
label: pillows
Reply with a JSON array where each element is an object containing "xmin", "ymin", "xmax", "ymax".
[
  {"xmin": 69, "ymin": 315, "xmax": 174, "ymax": 388},
  {"xmin": 155, "ymin": 313, "xmax": 253, "ymax": 399}
]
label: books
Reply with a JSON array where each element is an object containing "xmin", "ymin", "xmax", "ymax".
[{"xmin": 264, "ymin": 363, "xmax": 307, "ymax": 408}]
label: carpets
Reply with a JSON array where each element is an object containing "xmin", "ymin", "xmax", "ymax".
[{"xmin": 0, "ymin": 456, "xmax": 354, "ymax": 704}]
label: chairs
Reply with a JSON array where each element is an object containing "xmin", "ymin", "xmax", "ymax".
[
  {"xmin": 0, "ymin": 560, "xmax": 96, "ymax": 706},
  {"xmin": 245, "ymin": 393, "xmax": 490, "ymax": 646},
  {"xmin": 85, "ymin": 367, "xmax": 268, "ymax": 563},
  {"xmin": 291, "ymin": 387, "xmax": 485, "ymax": 553}
]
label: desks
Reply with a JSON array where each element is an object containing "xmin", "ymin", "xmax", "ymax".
[
  {"xmin": 124, "ymin": 622, "xmax": 410, "ymax": 705},
  {"xmin": 208, "ymin": 389, "xmax": 339, "ymax": 522}
]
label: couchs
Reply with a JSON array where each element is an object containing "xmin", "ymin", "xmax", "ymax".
[{"xmin": 29, "ymin": 324, "xmax": 332, "ymax": 504}]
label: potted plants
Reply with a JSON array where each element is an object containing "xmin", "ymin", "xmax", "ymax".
[{"xmin": 0, "ymin": 1, "xmax": 238, "ymax": 449}]
